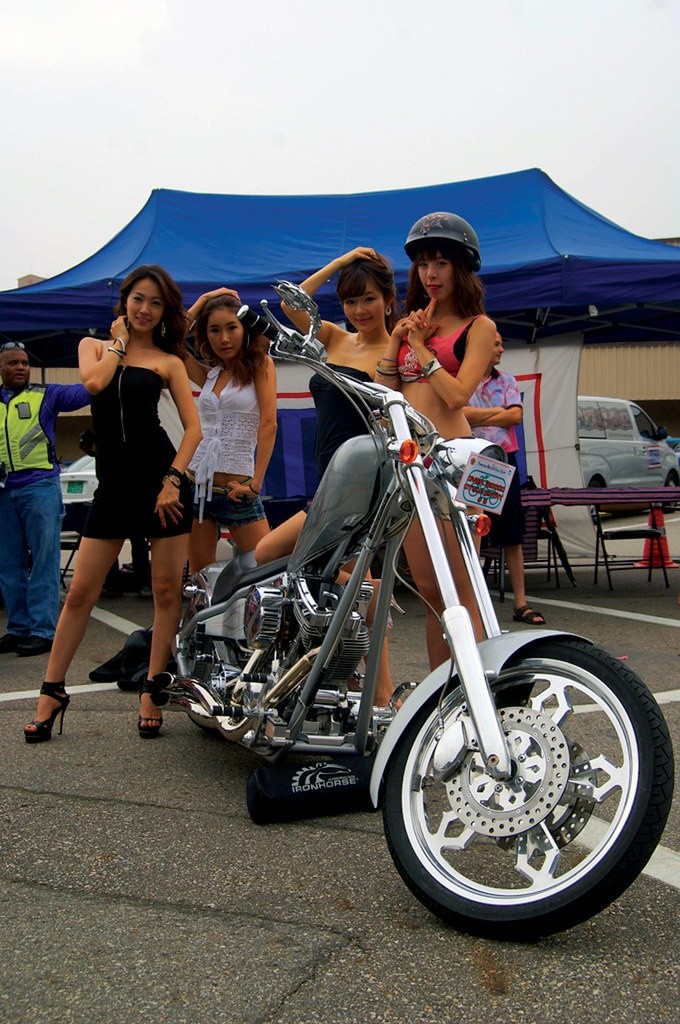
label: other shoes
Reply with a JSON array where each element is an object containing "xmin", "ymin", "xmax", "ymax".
[{"xmin": 0, "ymin": 633, "xmax": 53, "ymax": 656}]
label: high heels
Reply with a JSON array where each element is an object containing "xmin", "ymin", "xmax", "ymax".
[
  {"xmin": 23, "ymin": 680, "xmax": 71, "ymax": 744},
  {"xmin": 138, "ymin": 677, "xmax": 164, "ymax": 739}
]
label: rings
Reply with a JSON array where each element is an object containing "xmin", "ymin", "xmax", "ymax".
[
  {"xmin": 368, "ymin": 249, "xmax": 373, "ymax": 255},
  {"xmin": 113, "ymin": 321, "xmax": 117, "ymax": 324}
]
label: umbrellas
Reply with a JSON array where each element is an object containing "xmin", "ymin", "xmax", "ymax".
[
  {"xmin": 0, "ymin": 167, "xmax": 680, "ymax": 602},
  {"xmin": 526, "ymin": 473, "xmax": 578, "ymax": 588}
]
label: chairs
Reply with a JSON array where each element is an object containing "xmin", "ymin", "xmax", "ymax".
[
  {"xmin": 492, "ymin": 476, "xmax": 559, "ymax": 590},
  {"xmin": 594, "ymin": 502, "xmax": 670, "ymax": 591}
]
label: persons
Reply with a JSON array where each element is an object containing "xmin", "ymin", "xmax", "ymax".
[
  {"xmin": 0, "ymin": 342, "xmax": 92, "ymax": 656},
  {"xmin": 462, "ymin": 333, "xmax": 546, "ymax": 625},
  {"xmin": 23, "ymin": 265, "xmax": 203, "ymax": 744},
  {"xmin": 254, "ymin": 247, "xmax": 402, "ymax": 711},
  {"xmin": 373, "ymin": 211, "xmax": 497, "ymax": 672},
  {"xmin": 179, "ymin": 287, "xmax": 278, "ymax": 573}
]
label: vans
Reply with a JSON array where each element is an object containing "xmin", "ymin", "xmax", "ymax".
[{"xmin": 578, "ymin": 396, "xmax": 680, "ymax": 524}]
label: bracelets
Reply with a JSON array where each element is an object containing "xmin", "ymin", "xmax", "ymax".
[
  {"xmin": 248, "ymin": 484, "xmax": 261, "ymax": 495},
  {"xmin": 108, "ymin": 338, "xmax": 128, "ymax": 359},
  {"xmin": 376, "ymin": 358, "xmax": 399, "ymax": 382},
  {"xmin": 162, "ymin": 467, "xmax": 183, "ymax": 488},
  {"xmin": 422, "ymin": 358, "xmax": 442, "ymax": 378}
]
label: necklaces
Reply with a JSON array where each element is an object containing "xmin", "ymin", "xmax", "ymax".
[{"xmin": 224, "ymin": 365, "xmax": 232, "ymax": 376}]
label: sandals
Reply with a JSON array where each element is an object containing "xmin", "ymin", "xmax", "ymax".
[{"xmin": 512, "ymin": 605, "xmax": 546, "ymax": 625}]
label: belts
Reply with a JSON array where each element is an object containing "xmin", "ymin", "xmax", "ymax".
[{"xmin": 187, "ymin": 473, "xmax": 252, "ymax": 495}]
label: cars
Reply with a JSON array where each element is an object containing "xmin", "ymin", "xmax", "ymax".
[{"xmin": 58, "ymin": 454, "xmax": 98, "ymax": 518}]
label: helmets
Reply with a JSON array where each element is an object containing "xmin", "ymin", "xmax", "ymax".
[{"xmin": 404, "ymin": 212, "xmax": 482, "ymax": 271}]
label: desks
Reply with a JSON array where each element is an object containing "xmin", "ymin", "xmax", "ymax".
[
  {"xmin": 60, "ymin": 502, "xmax": 189, "ymax": 590},
  {"xmin": 482, "ymin": 485, "xmax": 678, "ymax": 603}
]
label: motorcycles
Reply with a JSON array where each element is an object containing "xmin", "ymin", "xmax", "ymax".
[{"xmin": 149, "ymin": 277, "xmax": 676, "ymax": 945}]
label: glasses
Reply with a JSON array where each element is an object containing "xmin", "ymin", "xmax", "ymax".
[{"xmin": 0, "ymin": 342, "xmax": 25, "ymax": 353}]
